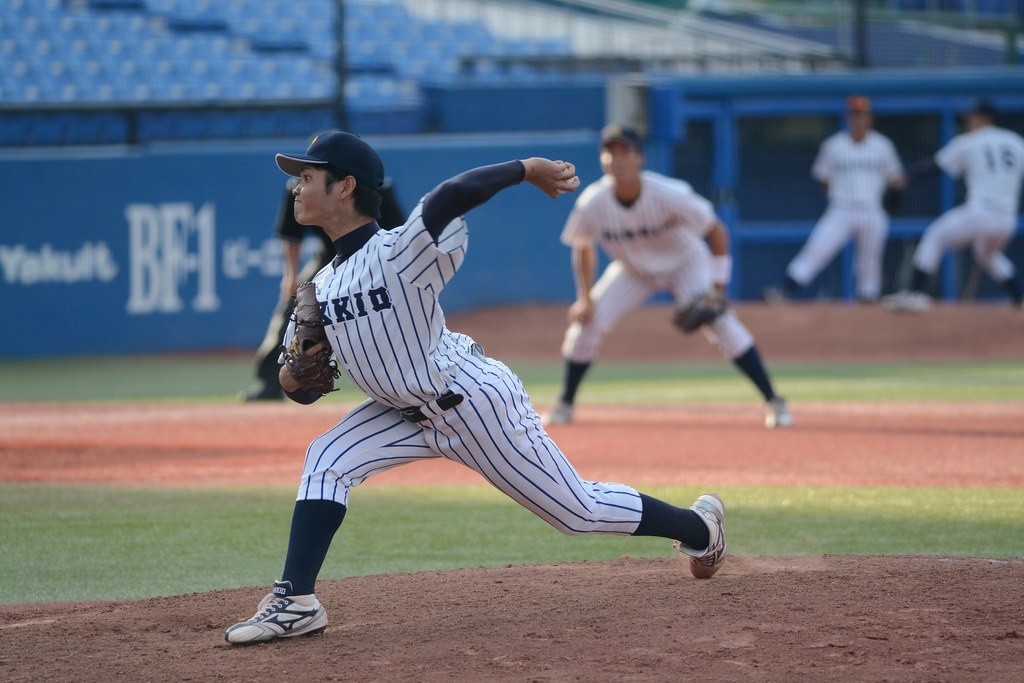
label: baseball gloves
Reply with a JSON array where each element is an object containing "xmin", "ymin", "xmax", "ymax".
[
  {"xmin": 279, "ymin": 281, "xmax": 341, "ymax": 396},
  {"xmin": 672, "ymin": 295, "xmax": 726, "ymax": 333}
]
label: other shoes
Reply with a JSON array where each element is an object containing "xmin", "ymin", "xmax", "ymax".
[
  {"xmin": 538, "ymin": 399, "xmax": 573, "ymax": 428},
  {"xmin": 765, "ymin": 398, "xmax": 790, "ymax": 427},
  {"xmin": 244, "ymin": 383, "xmax": 285, "ymax": 402},
  {"xmin": 881, "ymin": 287, "xmax": 934, "ymax": 315}
]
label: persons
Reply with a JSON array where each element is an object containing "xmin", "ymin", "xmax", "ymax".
[
  {"xmin": 224, "ymin": 131, "xmax": 728, "ymax": 645},
  {"xmin": 768, "ymin": 95, "xmax": 904, "ymax": 306},
  {"xmin": 238, "ymin": 176, "xmax": 405, "ymax": 403},
  {"xmin": 538, "ymin": 122, "xmax": 793, "ymax": 429},
  {"xmin": 879, "ymin": 97, "xmax": 1024, "ymax": 311}
]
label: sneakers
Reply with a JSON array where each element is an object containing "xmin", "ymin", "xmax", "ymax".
[
  {"xmin": 223, "ymin": 580, "xmax": 328, "ymax": 644},
  {"xmin": 673, "ymin": 494, "xmax": 728, "ymax": 579}
]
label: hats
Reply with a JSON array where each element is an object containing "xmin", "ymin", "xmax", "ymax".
[
  {"xmin": 846, "ymin": 96, "xmax": 871, "ymax": 115},
  {"xmin": 275, "ymin": 131, "xmax": 384, "ymax": 195},
  {"xmin": 599, "ymin": 122, "xmax": 644, "ymax": 151}
]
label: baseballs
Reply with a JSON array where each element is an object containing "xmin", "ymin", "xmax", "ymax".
[{"xmin": 555, "ymin": 167, "xmax": 575, "ymax": 194}]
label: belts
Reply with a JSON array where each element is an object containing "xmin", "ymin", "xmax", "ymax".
[{"xmin": 399, "ymin": 390, "xmax": 464, "ymax": 424}]
